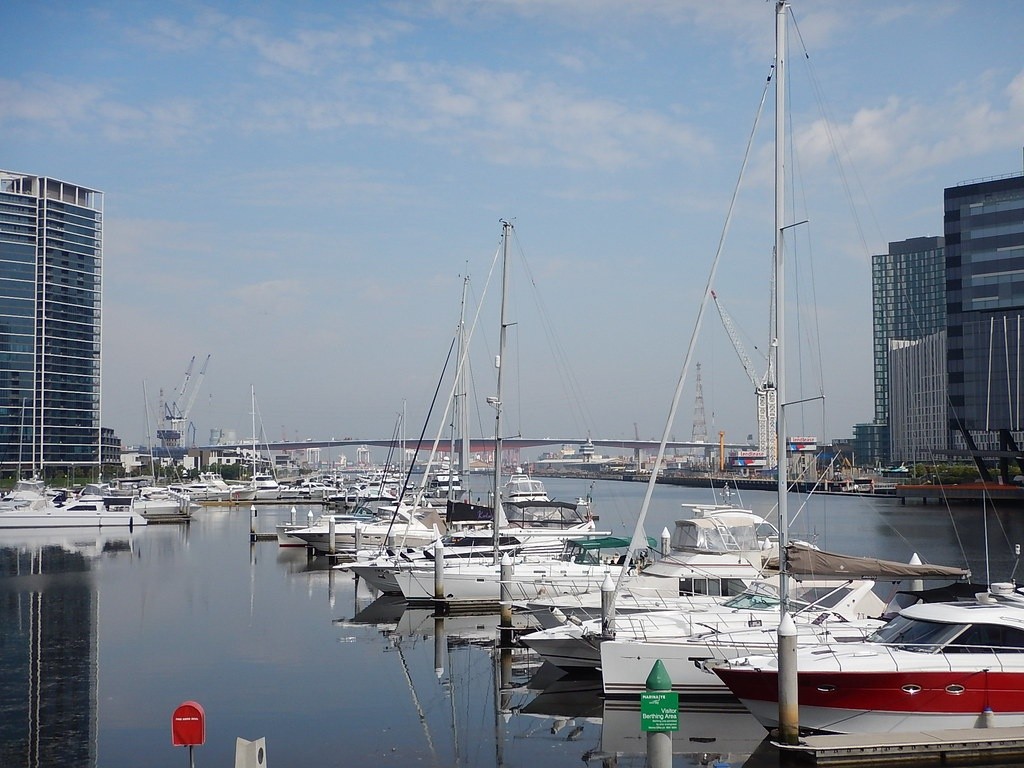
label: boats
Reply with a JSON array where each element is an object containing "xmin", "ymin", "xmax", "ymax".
[
  {"xmin": 702, "ymin": 582, "xmax": 1024, "ymax": 768},
  {"xmin": 0, "ymin": 526, "xmax": 147, "ymax": 561},
  {"xmin": 0, "ymin": 379, "xmax": 467, "ymax": 528}
]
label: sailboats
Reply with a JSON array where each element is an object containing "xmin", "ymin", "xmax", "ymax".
[
  {"xmin": 332, "ymin": 594, "xmax": 772, "ymax": 768},
  {"xmin": 275, "ymin": 0, "xmax": 1020, "ymax": 700}
]
